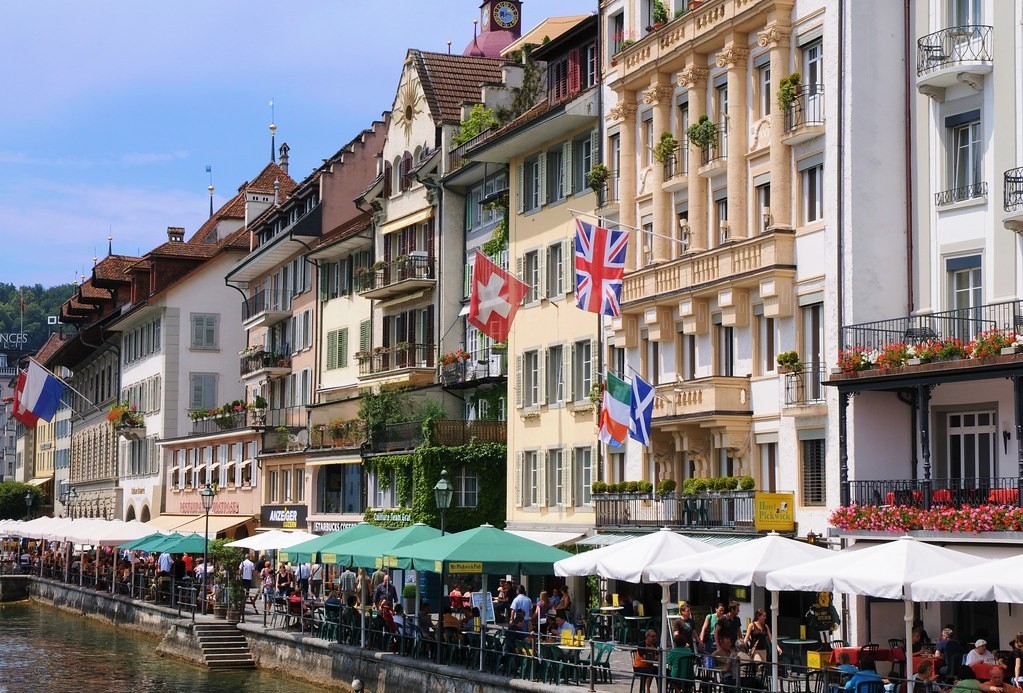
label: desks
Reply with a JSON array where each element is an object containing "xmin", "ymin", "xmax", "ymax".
[{"xmin": 989, "ymin": 489, "xmax": 1019, "ymax": 505}]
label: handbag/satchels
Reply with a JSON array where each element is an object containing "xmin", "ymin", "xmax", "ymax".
[
  {"xmin": 702, "ymin": 614, "xmax": 712, "ymax": 643},
  {"xmin": 540, "ymin": 617, "xmax": 547, "ymax": 624},
  {"xmin": 259, "ymin": 568, "xmax": 265, "ymax": 579}
]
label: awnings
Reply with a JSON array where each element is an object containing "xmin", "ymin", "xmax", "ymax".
[
  {"xmin": 145, "ymin": 513, "xmax": 254, "ymax": 535},
  {"xmin": 576, "ymin": 532, "xmax": 765, "ymax": 549},
  {"xmin": 840, "ymin": 540, "xmax": 1023, "ymax": 560},
  {"xmin": 504, "ymin": 530, "xmax": 587, "ymax": 547}
]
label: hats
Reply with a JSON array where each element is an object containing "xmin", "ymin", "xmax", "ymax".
[{"xmin": 975, "ymin": 639, "xmax": 987, "ymax": 648}]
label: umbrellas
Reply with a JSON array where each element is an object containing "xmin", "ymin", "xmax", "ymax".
[
  {"xmin": 223, "ymin": 521, "xmax": 577, "ymax": 671},
  {"xmin": 649, "ymin": 529, "xmax": 839, "ymax": 693},
  {"xmin": 554, "ymin": 525, "xmax": 720, "ymax": 693},
  {"xmin": 910, "ymin": 553, "xmax": 1023, "ymax": 604},
  {"xmin": 0, "ymin": 516, "xmax": 236, "ymax": 589}
]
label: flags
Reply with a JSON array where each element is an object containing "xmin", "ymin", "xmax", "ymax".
[
  {"xmin": 468, "ymin": 248, "xmax": 531, "ymax": 345},
  {"xmin": 20, "ymin": 359, "xmax": 67, "ymax": 423},
  {"xmin": 597, "ymin": 371, "xmax": 631, "ymax": 448},
  {"xmin": 12, "ymin": 370, "xmax": 40, "ymax": 430},
  {"xmin": 20, "ymin": 294, "xmax": 24, "ymax": 317},
  {"xmin": 630, "ymin": 373, "xmax": 655, "ymax": 447},
  {"xmin": 575, "ymin": 217, "xmax": 630, "ymax": 316}
]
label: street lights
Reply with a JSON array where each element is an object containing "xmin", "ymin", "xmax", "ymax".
[
  {"xmin": 433, "ymin": 468, "xmax": 455, "ymax": 662},
  {"xmin": 26, "ymin": 490, "xmax": 33, "ymax": 519},
  {"xmin": 200, "ymin": 482, "xmax": 215, "ymax": 612},
  {"xmin": 69, "ymin": 486, "xmax": 78, "ymax": 520}
]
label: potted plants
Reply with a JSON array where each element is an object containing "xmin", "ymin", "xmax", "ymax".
[
  {"xmin": 587, "ymin": 163, "xmax": 609, "ymax": 193},
  {"xmin": 777, "ymin": 72, "xmax": 802, "ymax": 111},
  {"xmin": 777, "ymin": 350, "xmax": 805, "ymax": 374},
  {"xmin": 651, "ymin": 0, "xmax": 667, "ymax": 30},
  {"xmin": 652, "ymin": 131, "xmax": 678, "ymax": 164},
  {"xmin": 592, "ymin": 477, "xmax": 755, "ymax": 500}
]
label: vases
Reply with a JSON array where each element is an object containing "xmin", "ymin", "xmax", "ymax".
[
  {"xmin": 442, "ymin": 362, "xmax": 461, "ymax": 373},
  {"xmin": 334, "ymin": 437, "xmax": 344, "ymax": 447},
  {"xmin": 688, "ymin": 0, "xmax": 705, "ymax": 10}
]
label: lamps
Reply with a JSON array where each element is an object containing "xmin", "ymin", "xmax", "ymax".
[
  {"xmin": 762, "ymin": 207, "xmax": 771, "ymax": 224},
  {"xmin": 807, "ymin": 530, "xmax": 822, "ymax": 543},
  {"xmin": 680, "ymin": 219, "xmax": 689, "ymax": 233},
  {"xmin": 1002, "ymin": 421, "xmax": 1011, "ymax": 455},
  {"xmin": 720, "ymin": 220, "xmax": 730, "ymax": 235}
]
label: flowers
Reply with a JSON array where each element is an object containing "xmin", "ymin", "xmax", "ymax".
[
  {"xmin": 827, "ymin": 503, "xmax": 1023, "ymax": 536},
  {"xmin": 327, "ymin": 416, "xmax": 348, "ymax": 437},
  {"xmin": 838, "ymin": 328, "xmax": 1019, "ymax": 374},
  {"xmin": 189, "ymin": 400, "xmax": 250, "ymax": 426},
  {"xmin": 106, "ymin": 400, "xmax": 144, "ymax": 428},
  {"xmin": 437, "ymin": 348, "xmax": 470, "ymax": 367}
]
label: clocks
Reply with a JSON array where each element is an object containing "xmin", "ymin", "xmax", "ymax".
[{"xmin": 493, "ymin": 1, "xmax": 518, "ymax": 29}]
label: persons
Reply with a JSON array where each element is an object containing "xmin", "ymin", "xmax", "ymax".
[
  {"xmin": 766, "ymin": 532, "xmax": 994, "ymax": 693},
  {"xmin": 0, "ymin": 536, "xmax": 229, "ymax": 613},
  {"xmin": 238, "ymin": 552, "xmax": 576, "ymax": 665},
  {"xmin": 835, "ymin": 620, "xmax": 1023, "ymax": 693},
  {"xmin": 623, "ymin": 592, "xmax": 782, "ymax": 693}
]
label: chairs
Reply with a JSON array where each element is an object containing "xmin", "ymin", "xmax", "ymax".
[
  {"xmin": 0, "ymin": 559, "xmax": 1010, "ymax": 693},
  {"xmin": 873, "ymin": 488, "xmax": 987, "ymax": 510}
]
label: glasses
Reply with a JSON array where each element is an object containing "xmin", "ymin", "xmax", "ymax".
[
  {"xmin": 649, "ymin": 634, "xmax": 656, "ymax": 637},
  {"xmin": 517, "ymin": 613, "xmax": 523, "ymax": 616}
]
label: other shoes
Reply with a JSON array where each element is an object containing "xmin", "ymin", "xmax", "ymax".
[
  {"xmin": 263, "ymin": 611, "xmax": 266, "ymax": 614},
  {"xmin": 267, "ymin": 611, "xmax": 271, "ymax": 614}
]
